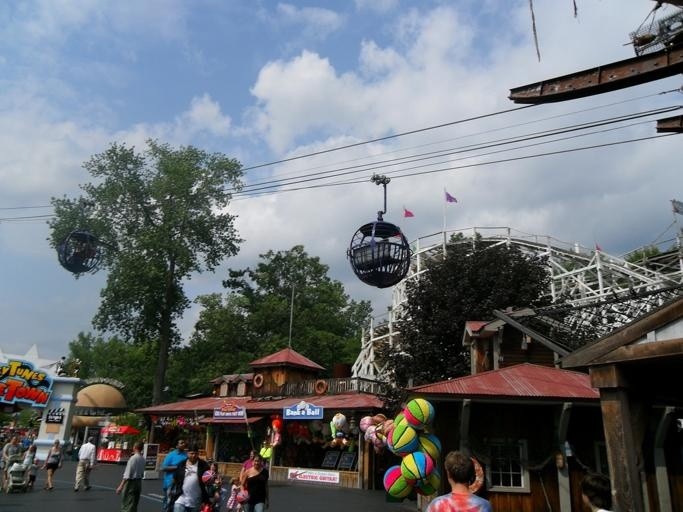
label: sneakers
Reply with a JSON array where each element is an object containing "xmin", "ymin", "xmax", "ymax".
[
  {"xmin": 43, "ymin": 485, "xmax": 54, "ymax": 490},
  {"xmin": 73, "ymin": 486, "xmax": 92, "ymax": 492}
]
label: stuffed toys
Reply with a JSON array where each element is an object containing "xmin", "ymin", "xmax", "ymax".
[{"xmin": 265, "ymin": 412, "xmax": 361, "ymax": 449}]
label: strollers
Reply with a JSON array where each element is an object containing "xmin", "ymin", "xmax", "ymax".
[{"xmin": 3, "ymin": 454, "xmax": 28, "ymax": 494}]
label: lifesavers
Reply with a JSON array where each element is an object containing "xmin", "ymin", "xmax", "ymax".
[
  {"xmin": 253, "ymin": 374, "xmax": 263, "ymax": 388},
  {"xmin": 469, "ymin": 458, "xmax": 484, "ymax": 493},
  {"xmin": 315, "ymin": 380, "xmax": 327, "ymax": 394}
]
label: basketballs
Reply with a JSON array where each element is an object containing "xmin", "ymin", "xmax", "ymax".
[
  {"xmin": 202, "ymin": 470, "xmax": 216, "ymax": 485},
  {"xmin": 383, "ymin": 398, "xmax": 441, "ymax": 499},
  {"xmin": 360, "ymin": 414, "xmax": 395, "ymax": 453},
  {"xmin": 237, "ymin": 491, "xmax": 250, "ymax": 504}
]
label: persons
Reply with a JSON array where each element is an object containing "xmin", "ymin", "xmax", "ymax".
[
  {"xmin": 579, "ymin": 472, "xmax": 614, "ymax": 512},
  {"xmin": 0, "ymin": 426, "xmax": 82, "ymax": 490},
  {"xmin": 114, "ymin": 439, "xmax": 146, "ymax": 511},
  {"xmin": 416, "ymin": 450, "xmax": 494, "ymax": 510},
  {"xmin": 106, "ymin": 438, "xmax": 115, "ymax": 449},
  {"xmin": 158, "ymin": 435, "xmax": 271, "ymax": 510},
  {"xmin": 72, "ymin": 436, "xmax": 98, "ymax": 492}
]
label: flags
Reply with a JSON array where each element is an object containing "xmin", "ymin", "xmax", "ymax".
[
  {"xmin": 445, "ymin": 192, "xmax": 459, "ymax": 204},
  {"xmin": 403, "ymin": 209, "xmax": 414, "ymax": 218}
]
